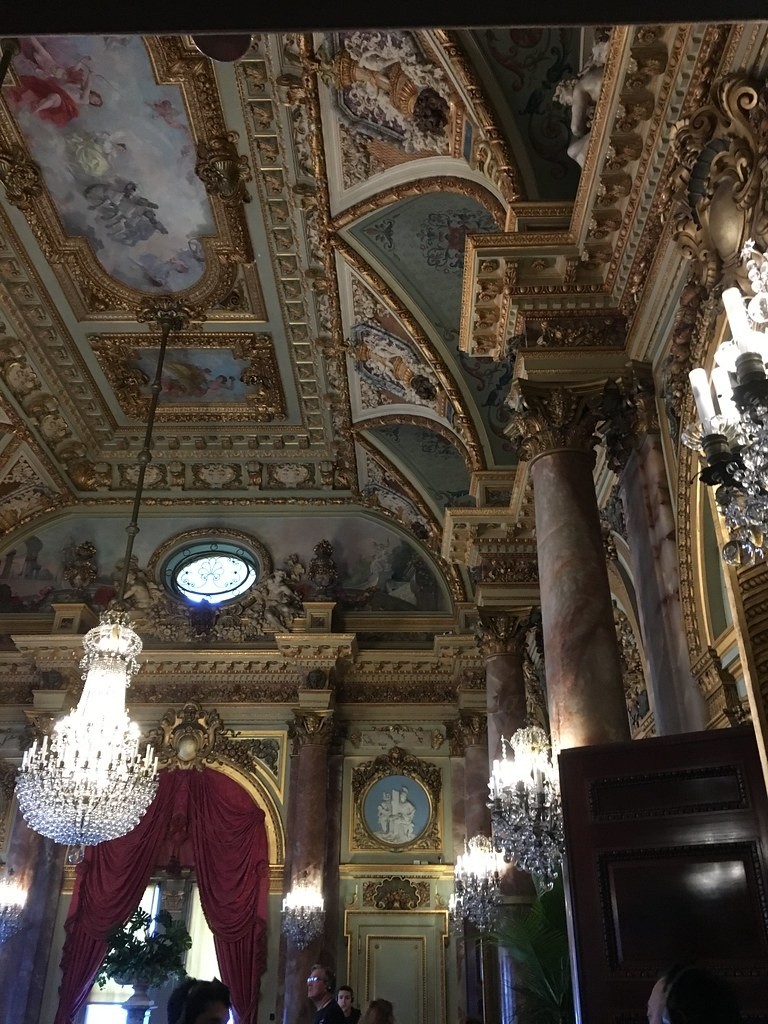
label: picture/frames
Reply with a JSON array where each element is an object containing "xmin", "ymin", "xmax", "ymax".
[{"xmin": 352, "ymin": 751, "xmax": 444, "ymax": 855}]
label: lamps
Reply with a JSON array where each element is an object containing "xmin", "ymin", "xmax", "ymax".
[
  {"xmin": 0, "ymin": 868, "xmax": 32, "ymax": 943},
  {"xmin": 446, "ymin": 835, "xmax": 513, "ymax": 933},
  {"xmin": 680, "ymin": 237, "xmax": 768, "ymax": 567},
  {"xmin": 16, "ymin": 296, "xmax": 206, "ymax": 861},
  {"xmin": 488, "ymin": 724, "xmax": 566, "ymax": 893},
  {"xmin": 282, "ymin": 883, "xmax": 328, "ymax": 951}
]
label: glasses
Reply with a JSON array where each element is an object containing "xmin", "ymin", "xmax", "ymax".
[{"xmin": 307, "ymin": 977, "xmax": 325, "ymax": 983}]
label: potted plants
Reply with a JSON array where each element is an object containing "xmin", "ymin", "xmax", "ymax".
[{"xmin": 95, "ymin": 909, "xmax": 194, "ymax": 1024}]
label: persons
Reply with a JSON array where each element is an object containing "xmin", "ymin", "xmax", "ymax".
[
  {"xmin": 552, "ymin": 65, "xmax": 608, "ymax": 171},
  {"xmin": 365, "ymin": 333, "xmax": 398, "ymax": 385},
  {"xmin": 110, "ymin": 555, "xmax": 153, "ymax": 626},
  {"xmin": 349, "ymin": 49, "xmax": 401, "ymax": 119},
  {"xmin": 307, "ymin": 964, "xmax": 398, "ymax": 1024},
  {"xmin": 251, "ymin": 571, "xmax": 304, "ymax": 633},
  {"xmin": 164, "ymin": 978, "xmax": 234, "ymax": 1024},
  {"xmin": 377, "ymin": 785, "xmax": 416, "ymax": 842},
  {"xmin": 646, "ymin": 964, "xmax": 744, "ymax": 1024}
]
